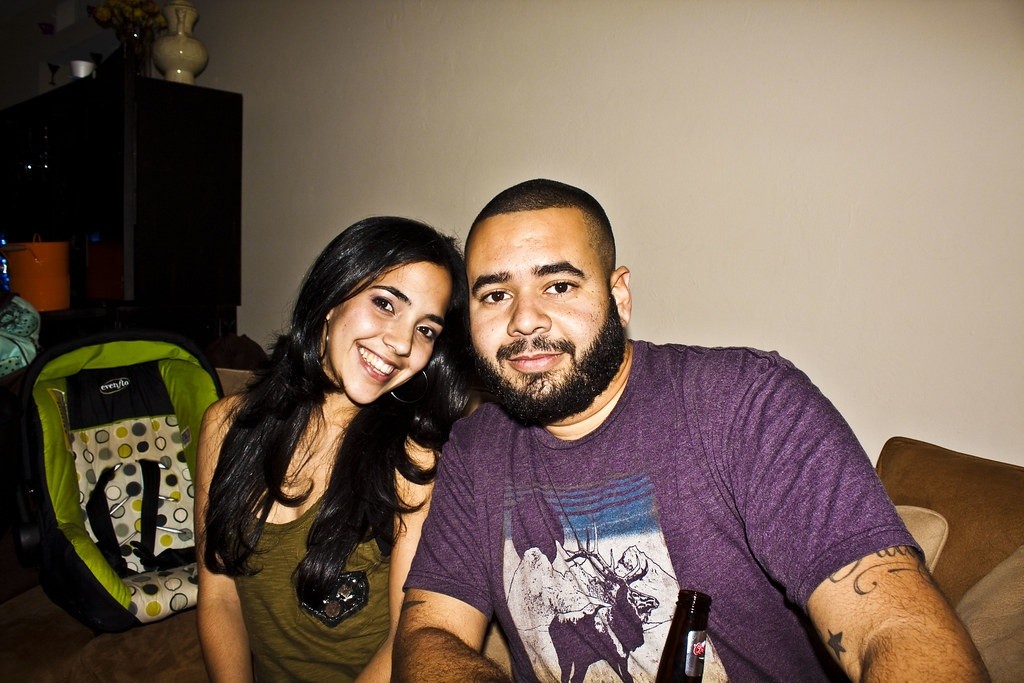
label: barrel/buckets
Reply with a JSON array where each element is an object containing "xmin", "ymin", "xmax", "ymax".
[{"xmin": 10, "ymin": 233, "xmax": 72, "ymax": 311}]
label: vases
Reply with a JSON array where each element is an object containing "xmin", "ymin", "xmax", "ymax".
[
  {"xmin": 120, "ymin": 25, "xmax": 156, "ymax": 78},
  {"xmin": 152, "ymin": 1, "xmax": 209, "ymax": 84}
]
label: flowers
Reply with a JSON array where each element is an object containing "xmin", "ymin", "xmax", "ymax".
[{"xmin": 85, "ymin": 0, "xmax": 167, "ymax": 78}]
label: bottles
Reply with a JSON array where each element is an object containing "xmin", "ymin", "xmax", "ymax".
[
  {"xmin": 151, "ymin": 0, "xmax": 208, "ymax": 86},
  {"xmin": 654, "ymin": 589, "xmax": 712, "ymax": 683}
]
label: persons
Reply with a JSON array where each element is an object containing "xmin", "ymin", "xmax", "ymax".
[
  {"xmin": 194, "ymin": 215, "xmax": 479, "ymax": 683},
  {"xmin": 391, "ymin": 178, "xmax": 993, "ymax": 683}
]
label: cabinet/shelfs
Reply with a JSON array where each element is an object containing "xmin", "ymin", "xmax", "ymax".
[{"xmin": 0, "ymin": 77, "xmax": 243, "ymax": 343}]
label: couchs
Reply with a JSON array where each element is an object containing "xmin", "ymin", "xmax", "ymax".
[{"xmin": 873, "ymin": 435, "xmax": 1024, "ymax": 683}]
